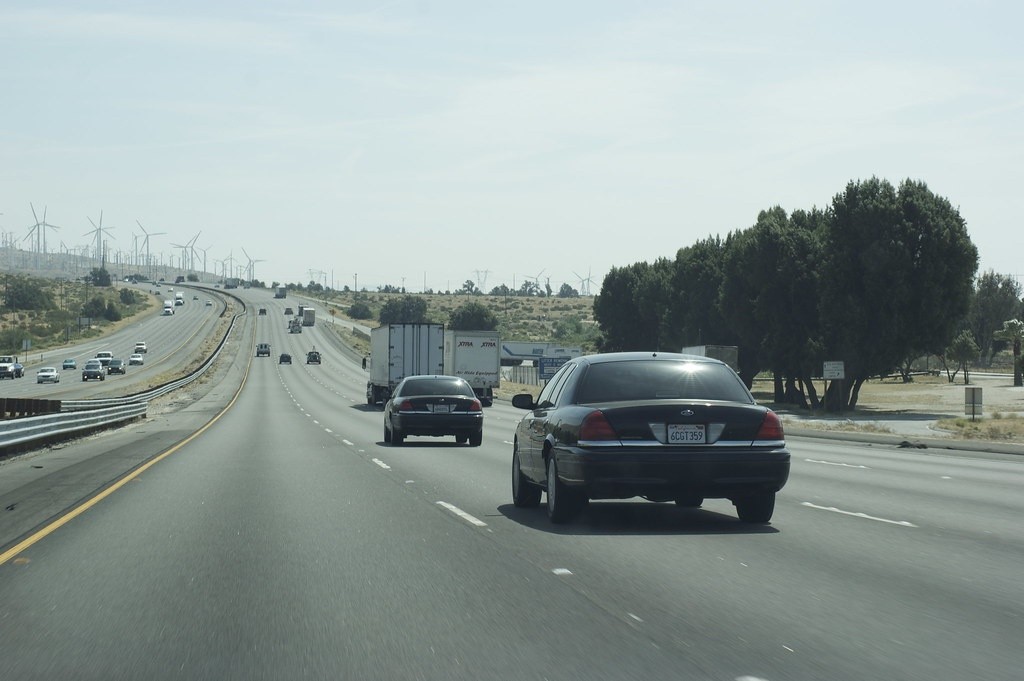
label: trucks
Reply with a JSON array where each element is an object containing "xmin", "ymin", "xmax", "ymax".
[
  {"xmin": 302, "ymin": 308, "xmax": 316, "ymax": 327},
  {"xmin": 361, "ymin": 321, "xmax": 503, "ymax": 408},
  {"xmin": 298, "ymin": 301, "xmax": 309, "ymax": 316}
]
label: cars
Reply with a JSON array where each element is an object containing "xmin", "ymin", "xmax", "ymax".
[
  {"xmin": 510, "ymin": 350, "xmax": 792, "ymax": 525},
  {"xmin": 81, "ymin": 358, "xmax": 106, "ymax": 382},
  {"xmin": 256, "ymin": 343, "xmax": 271, "ymax": 357},
  {"xmin": 62, "ymin": 358, "xmax": 77, "ymax": 370},
  {"xmin": 134, "ymin": 341, "xmax": 148, "ymax": 353},
  {"xmin": 94, "ymin": 351, "xmax": 114, "ymax": 366},
  {"xmin": 0, "ymin": 355, "xmax": 25, "ymax": 379},
  {"xmin": 129, "ymin": 354, "xmax": 144, "ymax": 366},
  {"xmin": 382, "ymin": 373, "xmax": 485, "ymax": 447},
  {"xmin": 124, "ymin": 274, "xmax": 304, "ymax": 334},
  {"xmin": 305, "ymin": 351, "xmax": 322, "ymax": 364},
  {"xmin": 107, "ymin": 359, "xmax": 127, "ymax": 375},
  {"xmin": 36, "ymin": 366, "xmax": 60, "ymax": 384},
  {"xmin": 278, "ymin": 353, "xmax": 292, "ymax": 364}
]
